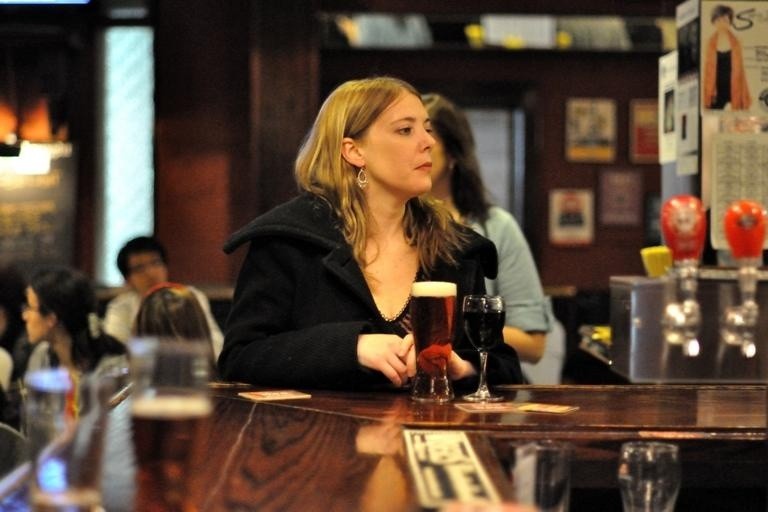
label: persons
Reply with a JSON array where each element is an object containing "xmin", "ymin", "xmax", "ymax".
[
  {"xmin": 9, "ymin": 264, "xmax": 140, "ymax": 511},
  {"xmin": 415, "ymin": 93, "xmax": 555, "ymax": 366},
  {"xmin": 104, "ymin": 236, "xmax": 226, "ymax": 367},
  {"xmin": 128, "ymin": 282, "xmax": 216, "ymax": 388},
  {"xmin": 217, "ymin": 76, "xmax": 523, "ymax": 392},
  {"xmin": 703, "ymin": 5, "xmax": 751, "ymax": 111},
  {"xmin": 0, "ymin": 264, "xmax": 35, "ymax": 432}
]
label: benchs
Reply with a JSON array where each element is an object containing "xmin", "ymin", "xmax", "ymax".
[{"xmin": 94, "ymin": 288, "xmax": 235, "ymax": 330}]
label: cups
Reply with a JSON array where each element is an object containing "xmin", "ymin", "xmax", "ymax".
[
  {"xmin": 618, "ymin": 441, "xmax": 683, "ymax": 512},
  {"xmin": 409, "ymin": 281, "xmax": 458, "ymax": 402},
  {"xmin": 25, "ymin": 363, "xmax": 108, "ymax": 512},
  {"xmin": 127, "ymin": 334, "xmax": 215, "ymax": 512}
]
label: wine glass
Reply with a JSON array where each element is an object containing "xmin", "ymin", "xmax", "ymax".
[{"xmin": 461, "ymin": 295, "xmax": 506, "ymax": 403}]
